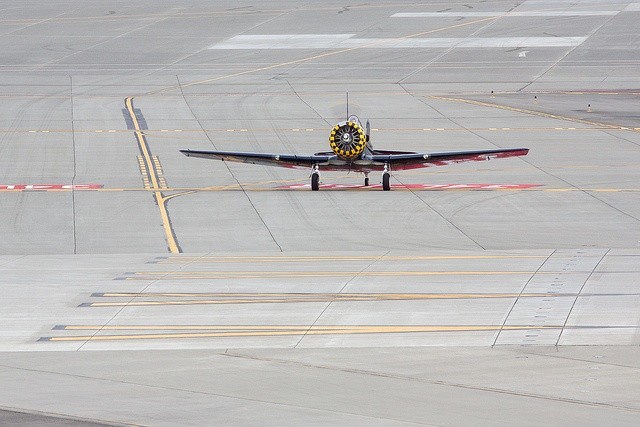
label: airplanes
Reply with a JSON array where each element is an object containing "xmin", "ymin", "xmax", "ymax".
[{"xmin": 178, "ymin": 91, "xmax": 529, "ymax": 191}]
